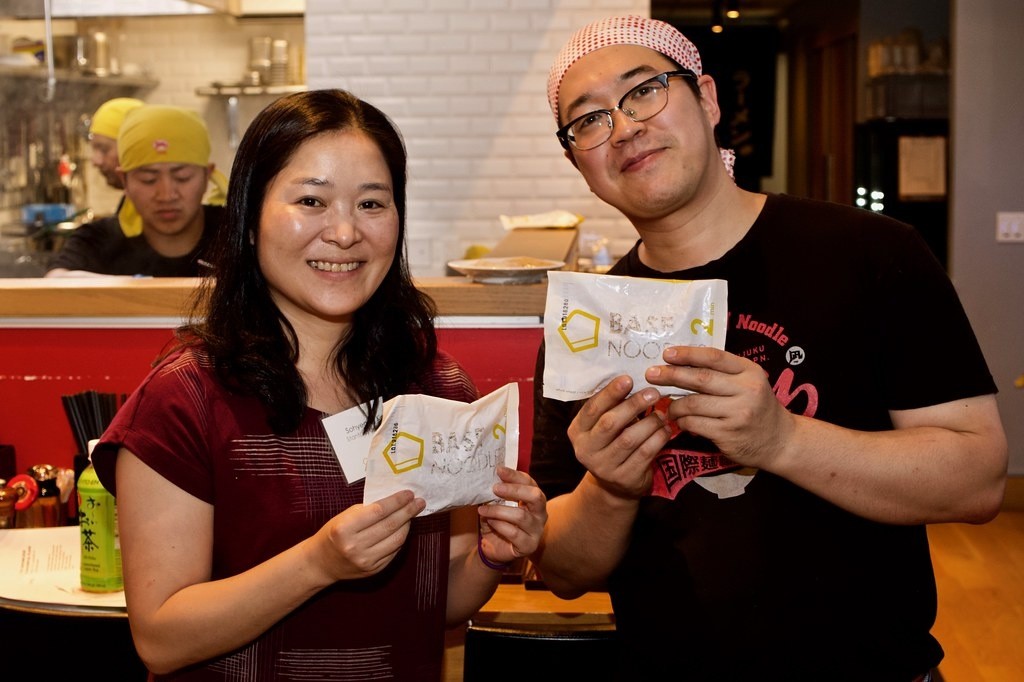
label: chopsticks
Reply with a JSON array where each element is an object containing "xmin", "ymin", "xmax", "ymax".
[{"xmin": 61, "ymin": 386, "xmax": 128, "ymax": 450}]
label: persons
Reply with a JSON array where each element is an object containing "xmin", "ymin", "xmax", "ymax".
[
  {"xmin": 87, "ymin": 95, "xmax": 148, "ymax": 216},
  {"xmin": 46, "ymin": 105, "xmax": 233, "ymax": 279},
  {"xmin": 93, "ymin": 88, "xmax": 548, "ymax": 682},
  {"xmin": 528, "ymin": 16, "xmax": 1011, "ymax": 682}
]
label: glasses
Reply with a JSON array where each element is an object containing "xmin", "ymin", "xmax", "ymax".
[{"xmin": 556, "ymin": 69, "xmax": 693, "ymax": 152}]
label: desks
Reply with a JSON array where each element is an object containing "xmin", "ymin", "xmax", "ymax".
[
  {"xmin": 444, "ymin": 583, "xmax": 617, "ymax": 682},
  {"xmin": 0, "ymin": 524, "xmax": 128, "ymax": 618}
]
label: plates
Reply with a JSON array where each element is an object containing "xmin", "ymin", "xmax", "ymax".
[{"xmin": 447, "ymin": 256, "xmax": 566, "ymax": 285}]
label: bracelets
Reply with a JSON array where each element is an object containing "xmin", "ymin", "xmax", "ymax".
[{"xmin": 477, "ymin": 536, "xmax": 512, "ymax": 571}]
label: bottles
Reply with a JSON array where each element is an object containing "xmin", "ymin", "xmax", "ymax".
[
  {"xmin": 32, "ymin": 464, "xmax": 61, "ymax": 527},
  {"xmin": 77, "ymin": 439, "xmax": 125, "ymax": 593}
]
